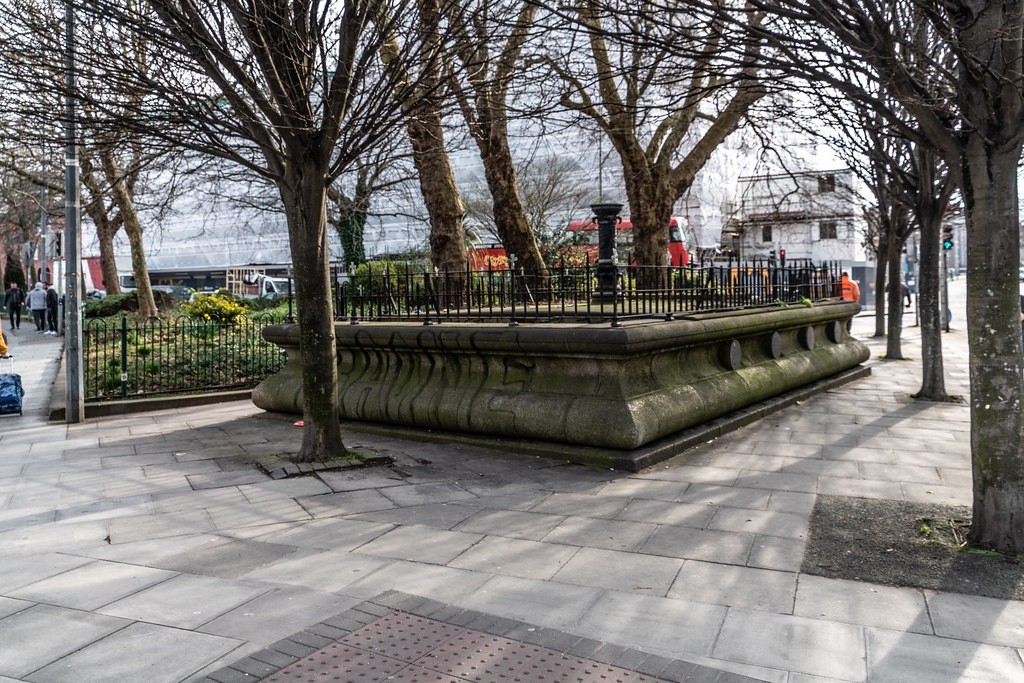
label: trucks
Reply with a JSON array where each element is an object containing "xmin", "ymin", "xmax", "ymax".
[{"xmin": 241, "ymin": 276, "xmax": 296, "ymax": 300}]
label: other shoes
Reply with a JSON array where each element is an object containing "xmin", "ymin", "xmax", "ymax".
[
  {"xmin": 44, "ymin": 330, "xmax": 51, "ymax": 335},
  {"xmin": 10, "ymin": 327, "xmax": 15, "ymax": 330},
  {"xmin": 17, "ymin": 327, "xmax": 20, "ymax": 329},
  {"xmin": 50, "ymin": 330, "xmax": 57, "ymax": 335}
]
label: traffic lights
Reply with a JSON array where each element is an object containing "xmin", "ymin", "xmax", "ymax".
[
  {"xmin": 780, "ymin": 250, "xmax": 785, "ymax": 265},
  {"xmin": 943, "ymin": 223, "xmax": 953, "ymax": 249}
]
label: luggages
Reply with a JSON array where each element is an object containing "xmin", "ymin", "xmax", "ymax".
[{"xmin": 0, "ymin": 355, "xmax": 25, "ymax": 416}]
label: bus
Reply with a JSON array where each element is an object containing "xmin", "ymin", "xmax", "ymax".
[{"xmin": 552, "ymin": 217, "xmax": 701, "ymax": 270}]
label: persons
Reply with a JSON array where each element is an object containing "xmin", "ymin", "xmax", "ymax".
[
  {"xmin": 24, "ymin": 282, "xmax": 47, "ymax": 332},
  {"xmin": 886, "ymin": 280, "xmax": 912, "ymax": 335},
  {"xmin": 841, "ymin": 272, "xmax": 860, "ymax": 333},
  {"xmin": 3, "ymin": 282, "xmax": 25, "ymax": 330},
  {"xmin": 0, "ymin": 318, "xmax": 12, "ymax": 359},
  {"xmin": 43, "ymin": 282, "xmax": 59, "ymax": 335}
]
